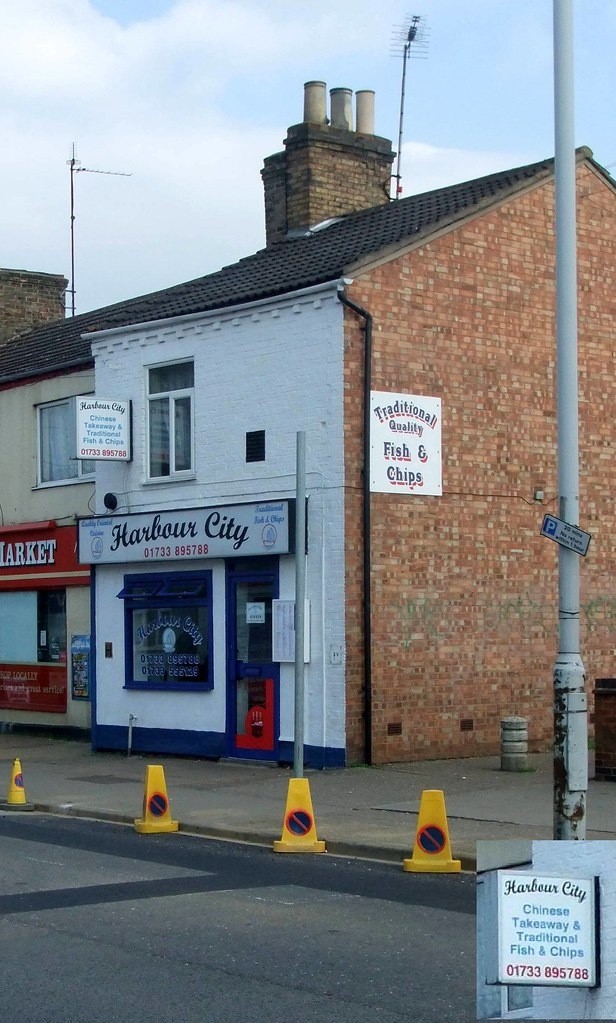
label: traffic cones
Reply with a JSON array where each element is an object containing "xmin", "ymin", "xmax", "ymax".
[
  {"xmin": 0, "ymin": 756, "xmax": 37, "ymax": 812},
  {"xmin": 271, "ymin": 778, "xmax": 326, "ymax": 853},
  {"xmin": 401, "ymin": 789, "xmax": 461, "ymax": 874},
  {"xmin": 131, "ymin": 765, "xmax": 179, "ymax": 832}
]
